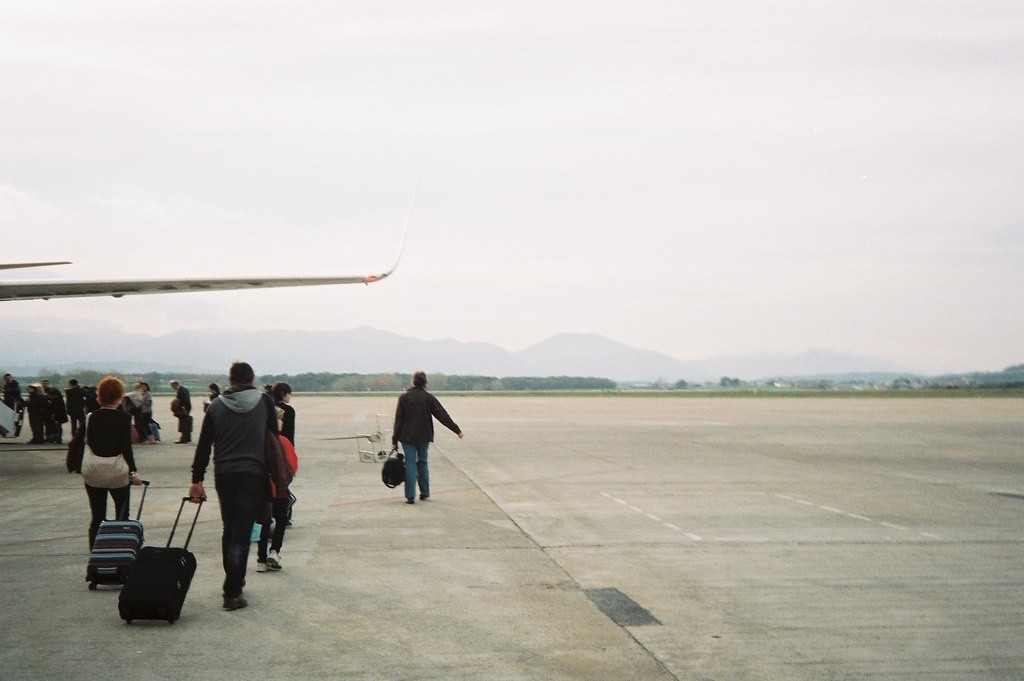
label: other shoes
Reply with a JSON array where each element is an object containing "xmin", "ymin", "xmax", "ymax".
[
  {"xmin": 420, "ymin": 493, "xmax": 426, "ymax": 500},
  {"xmin": 175, "ymin": 440, "xmax": 185, "ymax": 443},
  {"xmin": 223, "ymin": 593, "xmax": 247, "ymax": 608},
  {"xmin": 256, "ymin": 563, "xmax": 269, "ymax": 572},
  {"xmin": 405, "ymin": 498, "xmax": 414, "ymax": 503},
  {"xmin": 186, "ymin": 440, "xmax": 191, "ymax": 442},
  {"xmin": 267, "ymin": 549, "xmax": 282, "ymax": 569}
]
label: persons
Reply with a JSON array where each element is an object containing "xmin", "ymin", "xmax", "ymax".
[
  {"xmin": 254, "ymin": 381, "xmax": 296, "ymax": 573},
  {"xmin": 391, "ymin": 371, "xmax": 464, "ymax": 504},
  {"xmin": 0, "ymin": 373, "xmax": 271, "ymax": 445},
  {"xmin": 188, "ymin": 363, "xmax": 279, "ymax": 612},
  {"xmin": 76, "ymin": 376, "xmax": 139, "ymax": 553}
]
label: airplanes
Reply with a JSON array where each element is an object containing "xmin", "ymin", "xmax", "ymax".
[{"xmin": 0, "ymin": 258, "xmax": 402, "ymax": 303}]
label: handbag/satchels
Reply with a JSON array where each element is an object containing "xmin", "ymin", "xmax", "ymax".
[
  {"xmin": 82, "ymin": 444, "xmax": 131, "ymax": 488},
  {"xmin": 382, "ymin": 448, "xmax": 405, "ymax": 488},
  {"xmin": 178, "ymin": 415, "xmax": 193, "ymax": 432}
]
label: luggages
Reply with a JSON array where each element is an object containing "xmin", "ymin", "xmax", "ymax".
[
  {"xmin": 148, "ymin": 421, "xmax": 160, "ymax": 441},
  {"xmin": 119, "ymin": 496, "xmax": 205, "ymax": 624},
  {"xmin": 86, "ymin": 480, "xmax": 150, "ymax": 590}
]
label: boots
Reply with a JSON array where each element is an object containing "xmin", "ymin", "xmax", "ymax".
[{"xmin": 144, "ymin": 435, "xmax": 156, "ymax": 444}]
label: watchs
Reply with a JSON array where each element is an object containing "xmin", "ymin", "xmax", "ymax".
[{"xmin": 192, "ymin": 481, "xmax": 203, "ymax": 484}]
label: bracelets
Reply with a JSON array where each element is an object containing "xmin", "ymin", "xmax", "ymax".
[{"xmin": 129, "ymin": 471, "xmax": 139, "ymax": 478}]
